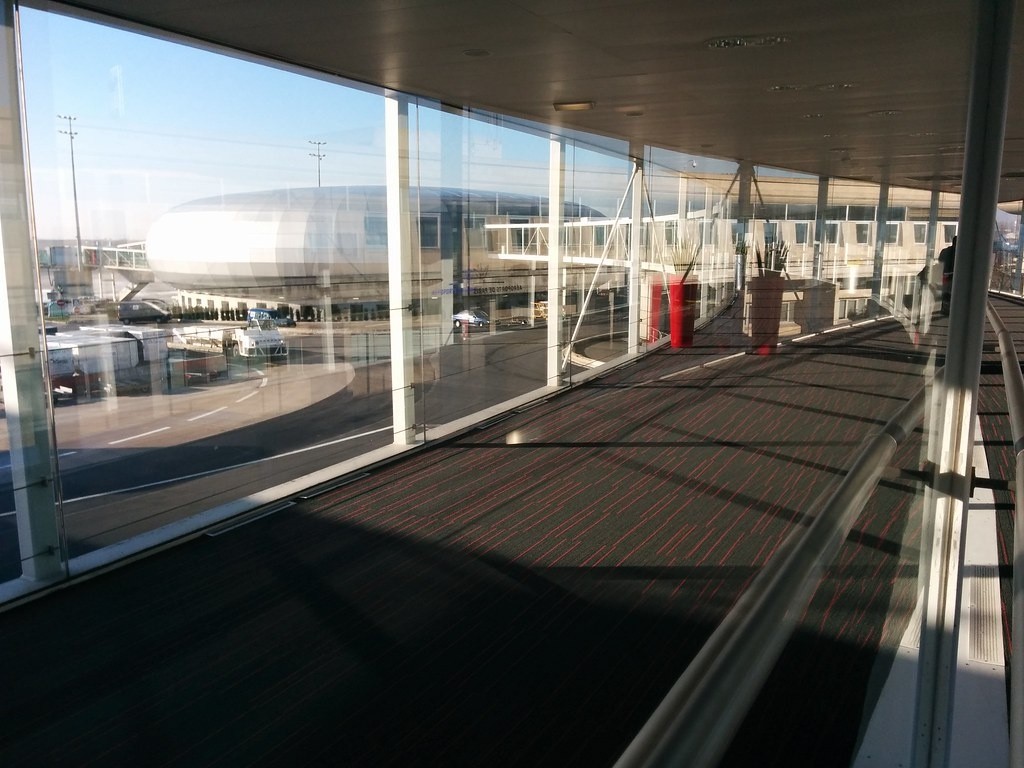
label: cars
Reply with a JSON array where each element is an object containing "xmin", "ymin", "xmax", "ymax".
[{"xmin": 451, "ymin": 310, "xmax": 501, "ymax": 328}]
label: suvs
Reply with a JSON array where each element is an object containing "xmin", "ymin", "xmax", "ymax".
[{"xmin": 247, "ymin": 308, "xmax": 297, "ymax": 327}]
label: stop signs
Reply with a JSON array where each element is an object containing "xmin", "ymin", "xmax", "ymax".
[{"xmin": 57, "ymin": 300, "xmax": 65, "ymax": 307}]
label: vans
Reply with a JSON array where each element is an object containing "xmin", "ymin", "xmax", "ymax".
[{"xmin": 117, "ymin": 299, "xmax": 173, "ymax": 323}]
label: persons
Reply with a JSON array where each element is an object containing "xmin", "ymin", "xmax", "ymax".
[{"xmin": 938, "ymin": 235, "xmax": 957, "ymax": 316}]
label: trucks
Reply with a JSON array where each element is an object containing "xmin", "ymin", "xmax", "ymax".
[{"xmin": 167, "ymin": 312, "xmax": 287, "ymax": 361}]
